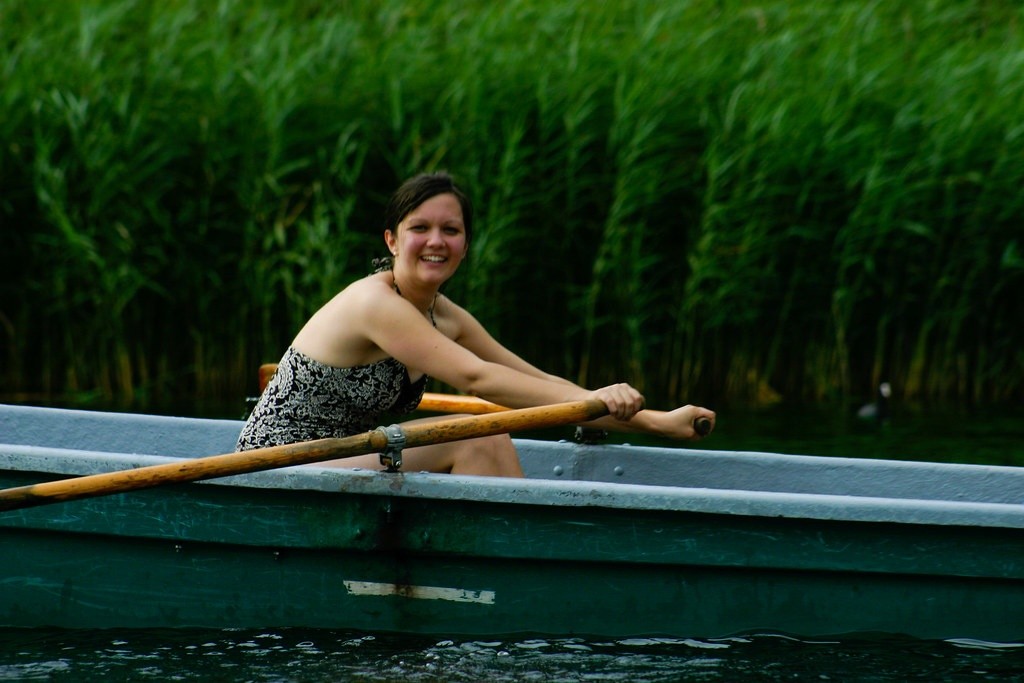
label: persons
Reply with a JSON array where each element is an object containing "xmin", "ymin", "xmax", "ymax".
[{"xmin": 236, "ymin": 173, "xmax": 717, "ymax": 477}]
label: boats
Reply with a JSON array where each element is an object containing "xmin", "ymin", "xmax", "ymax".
[{"xmin": 0, "ymin": 400, "xmax": 1024, "ymax": 652}]
